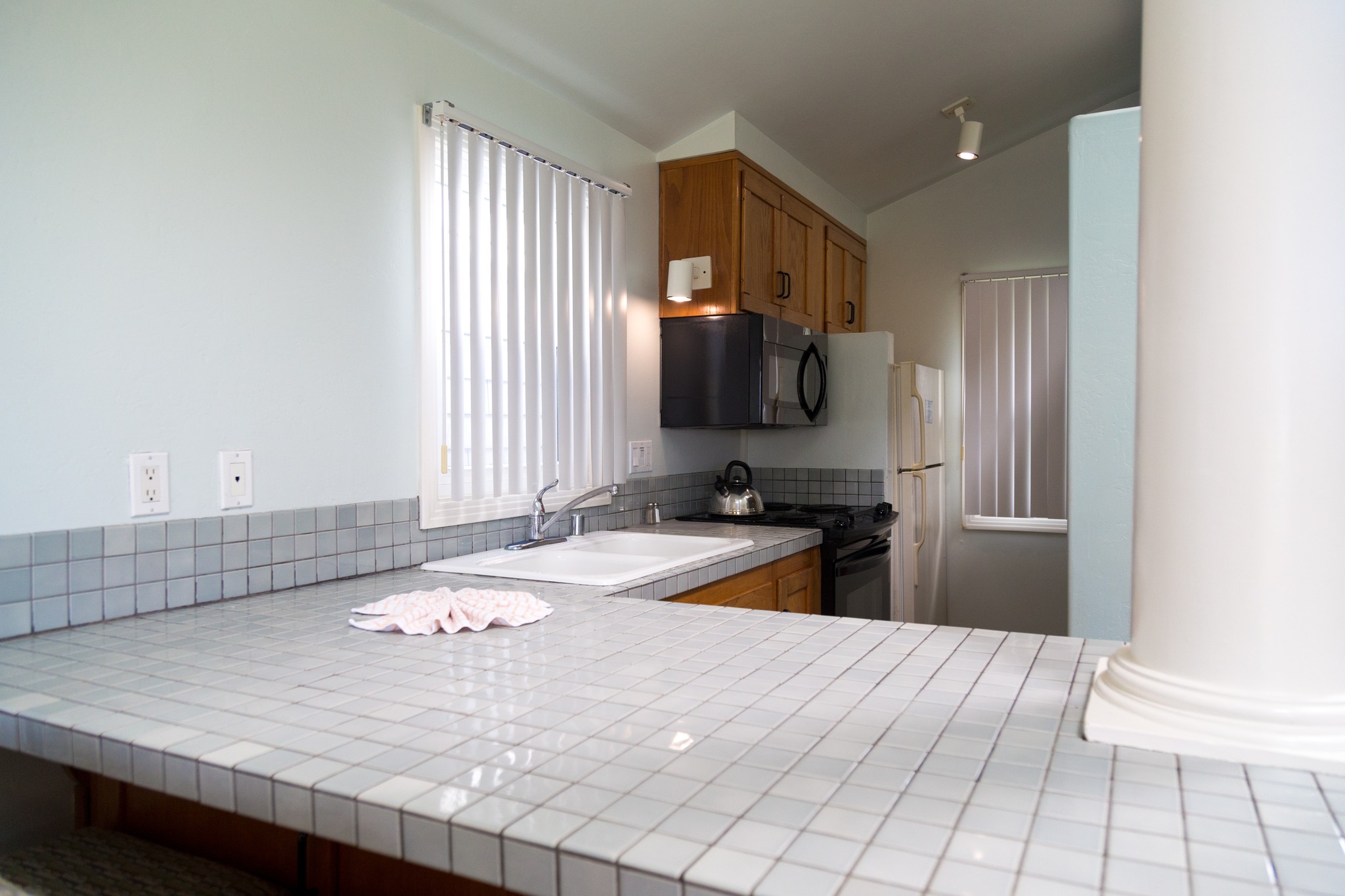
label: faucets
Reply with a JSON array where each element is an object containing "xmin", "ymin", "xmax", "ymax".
[{"xmin": 527, "ymin": 479, "xmax": 620, "ymax": 540}]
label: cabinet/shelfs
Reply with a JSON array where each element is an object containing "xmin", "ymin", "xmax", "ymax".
[
  {"xmin": 657, "ymin": 149, "xmax": 820, "ymax": 330},
  {"xmin": 772, "ymin": 545, "xmax": 822, "ymax": 615},
  {"xmin": 819, "ymin": 208, "xmax": 867, "ymax": 334}
]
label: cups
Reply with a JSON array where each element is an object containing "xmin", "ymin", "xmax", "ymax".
[{"xmin": 570, "ymin": 514, "xmax": 584, "ymax": 538}]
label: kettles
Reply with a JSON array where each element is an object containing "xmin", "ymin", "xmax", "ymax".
[{"xmin": 707, "ymin": 460, "xmax": 767, "ymax": 516}]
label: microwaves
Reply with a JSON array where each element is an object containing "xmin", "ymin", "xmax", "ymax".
[{"xmin": 660, "ymin": 312, "xmax": 830, "ymax": 431}]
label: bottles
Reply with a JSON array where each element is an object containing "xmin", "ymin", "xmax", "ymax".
[
  {"xmin": 645, "ymin": 502, "xmax": 656, "ymax": 524},
  {"xmin": 654, "ymin": 502, "xmax": 660, "ymax": 524}
]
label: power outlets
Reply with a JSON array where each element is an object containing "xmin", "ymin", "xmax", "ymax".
[{"xmin": 130, "ymin": 452, "xmax": 169, "ymax": 517}]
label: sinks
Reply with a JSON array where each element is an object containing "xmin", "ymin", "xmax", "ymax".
[{"xmin": 420, "ymin": 530, "xmax": 755, "ymax": 587}]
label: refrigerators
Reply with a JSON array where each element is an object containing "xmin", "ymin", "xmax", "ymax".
[{"xmin": 894, "ymin": 361, "xmax": 950, "ymax": 625}]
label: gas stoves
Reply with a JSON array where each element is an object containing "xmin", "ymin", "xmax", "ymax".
[{"xmin": 674, "ymin": 500, "xmax": 899, "ymax": 560}]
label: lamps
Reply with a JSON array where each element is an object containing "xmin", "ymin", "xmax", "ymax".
[
  {"xmin": 942, "ymin": 97, "xmax": 983, "ymax": 160},
  {"xmin": 667, "ymin": 255, "xmax": 713, "ymax": 303}
]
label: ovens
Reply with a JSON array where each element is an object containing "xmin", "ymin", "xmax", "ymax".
[{"xmin": 820, "ymin": 539, "xmax": 891, "ymax": 623}]
